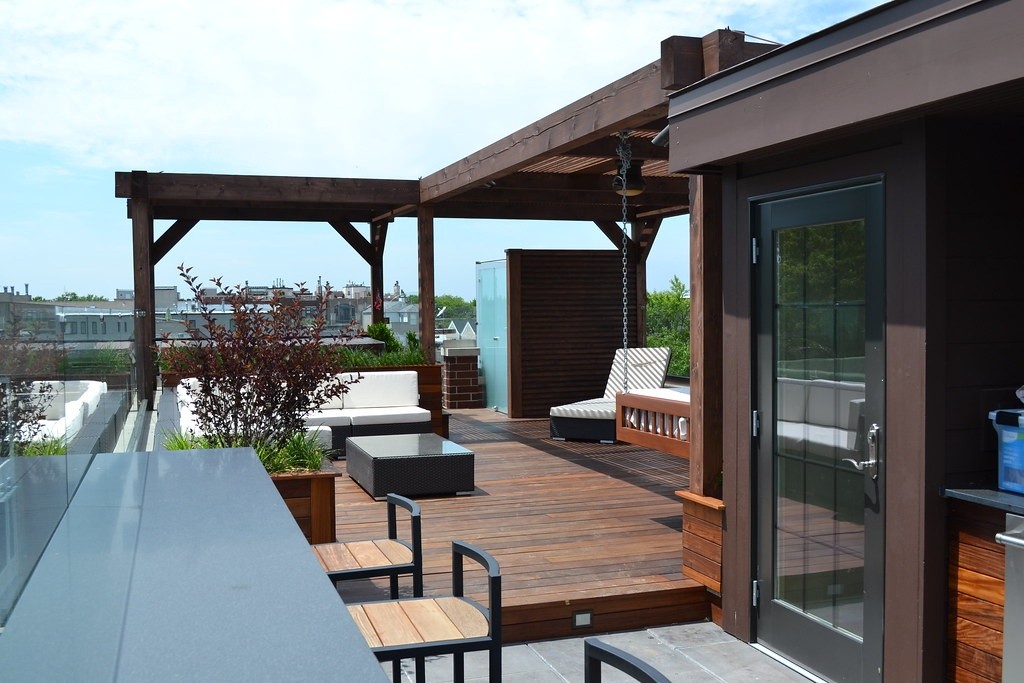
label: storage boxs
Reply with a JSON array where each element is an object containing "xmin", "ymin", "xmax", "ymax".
[{"xmin": 988, "ymin": 408, "xmax": 1023, "ymax": 494}]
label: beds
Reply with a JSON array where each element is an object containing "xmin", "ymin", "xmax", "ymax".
[{"xmin": 615, "ymin": 383, "xmax": 693, "ymax": 467}]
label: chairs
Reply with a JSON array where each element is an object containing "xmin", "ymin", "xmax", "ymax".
[
  {"xmin": 347, "ymin": 541, "xmax": 503, "ymax": 683},
  {"xmin": 308, "ymin": 491, "xmax": 423, "ymax": 599},
  {"xmin": 550, "ymin": 345, "xmax": 671, "ymax": 444}
]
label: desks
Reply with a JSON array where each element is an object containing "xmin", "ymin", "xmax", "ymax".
[{"xmin": 0, "ymin": 446, "xmax": 392, "ymax": 683}]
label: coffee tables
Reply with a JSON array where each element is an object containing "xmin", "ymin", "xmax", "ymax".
[{"xmin": 346, "ymin": 433, "xmax": 474, "ymax": 500}]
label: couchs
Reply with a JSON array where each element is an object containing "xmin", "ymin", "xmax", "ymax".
[
  {"xmin": 776, "ymin": 375, "xmax": 869, "ymax": 513},
  {"xmin": 178, "ymin": 369, "xmax": 431, "ymax": 452}
]
